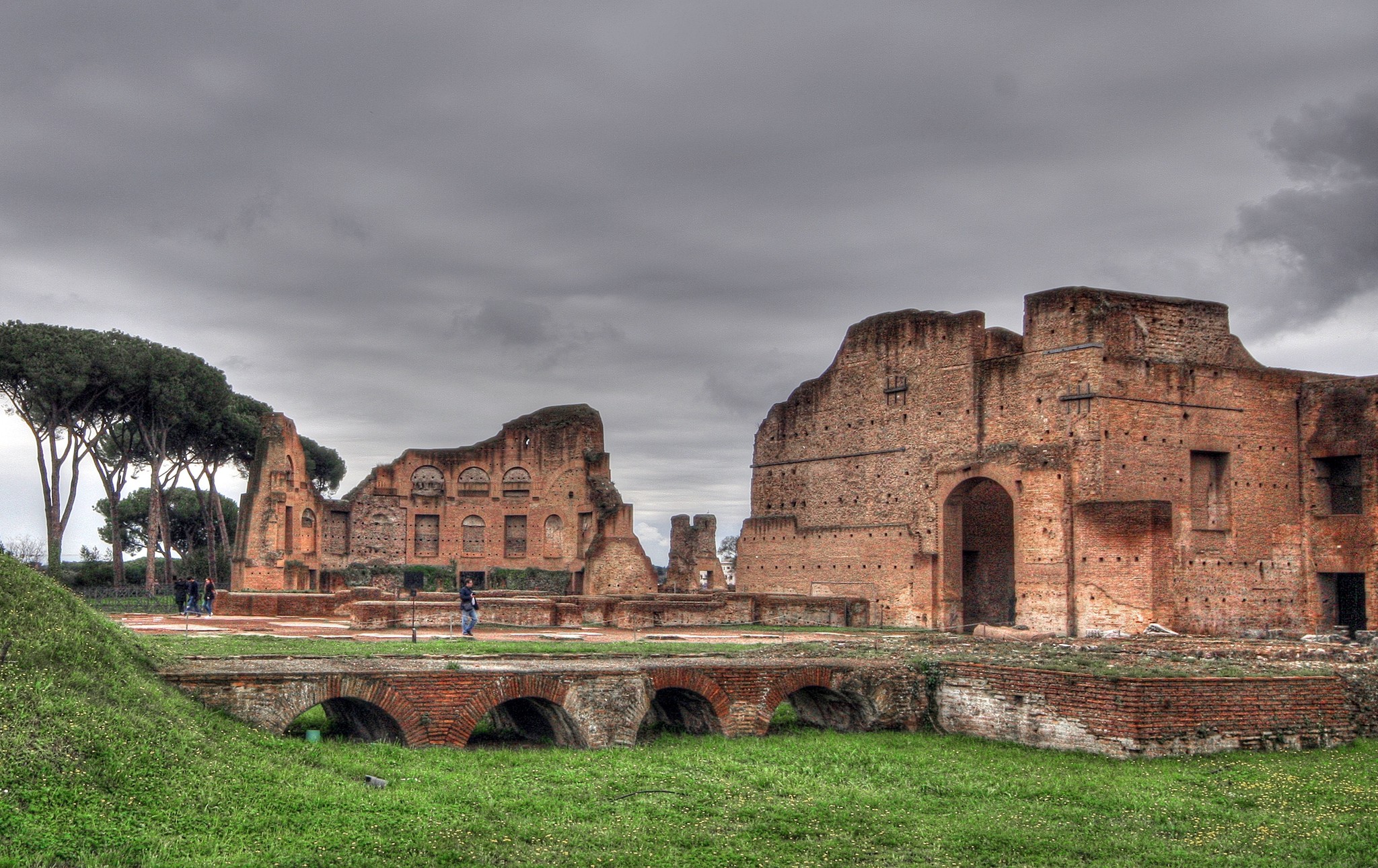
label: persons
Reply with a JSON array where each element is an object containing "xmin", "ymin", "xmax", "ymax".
[
  {"xmin": 174, "ymin": 577, "xmax": 189, "ymax": 617},
  {"xmin": 459, "ymin": 578, "xmax": 479, "ymax": 636},
  {"xmin": 201, "ymin": 577, "xmax": 218, "ymax": 616},
  {"xmin": 180, "ymin": 576, "xmax": 202, "ymax": 617}
]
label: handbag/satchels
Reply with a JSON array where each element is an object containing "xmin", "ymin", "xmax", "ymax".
[{"xmin": 462, "ymin": 603, "xmax": 473, "ymax": 612}]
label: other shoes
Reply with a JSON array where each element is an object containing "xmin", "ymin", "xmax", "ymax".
[
  {"xmin": 180, "ymin": 612, "xmax": 186, "ymax": 617},
  {"xmin": 467, "ymin": 631, "xmax": 472, "ymax": 636},
  {"xmin": 196, "ymin": 614, "xmax": 201, "ymax": 617},
  {"xmin": 463, "ymin": 633, "xmax": 468, "ymax": 635}
]
